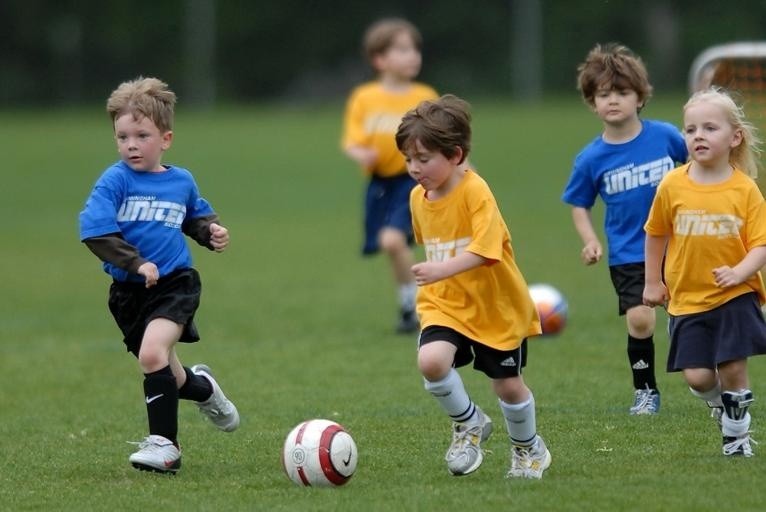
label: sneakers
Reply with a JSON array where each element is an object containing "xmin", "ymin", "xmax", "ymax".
[
  {"xmin": 190, "ymin": 364, "xmax": 240, "ymax": 432},
  {"xmin": 707, "ymin": 400, "xmax": 724, "ymax": 430},
  {"xmin": 445, "ymin": 404, "xmax": 494, "ymax": 476},
  {"xmin": 128, "ymin": 435, "xmax": 182, "ymax": 475},
  {"xmin": 721, "ymin": 390, "xmax": 753, "ymax": 457},
  {"xmin": 630, "ymin": 388, "xmax": 660, "ymax": 419},
  {"xmin": 398, "ymin": 296, "xmax": 417, "ymax": 332},
  {"xmin": 505, "ymin": 434, "xmax": 552, "ymax": 480}
]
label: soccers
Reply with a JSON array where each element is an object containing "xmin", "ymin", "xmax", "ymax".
[
  {"xmin": 283, "ymin": 418, "xmax": 359, "ymax": 490},
  {"xmin": 527, "ymin": 283, "xmax": 570, "ymax": 336}
]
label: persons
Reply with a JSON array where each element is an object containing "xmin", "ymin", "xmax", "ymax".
[
  {"xmin": 342, "ymin": 19, "xmax": 445, "ymax": 332},
  {"xmin": 79, "ymin": 79, "xmax": 239, "ymax": 474},
  {"xmin": 395, "ymin": 94, "xmax": 550, "ymax": 479},
  {"xmin": 560, "ymin": 45, "xmax": 691, "ymax": 415},
  {"xmin": 643, "ymin": 86, "xmax": 765, "ymax": 458}
]
